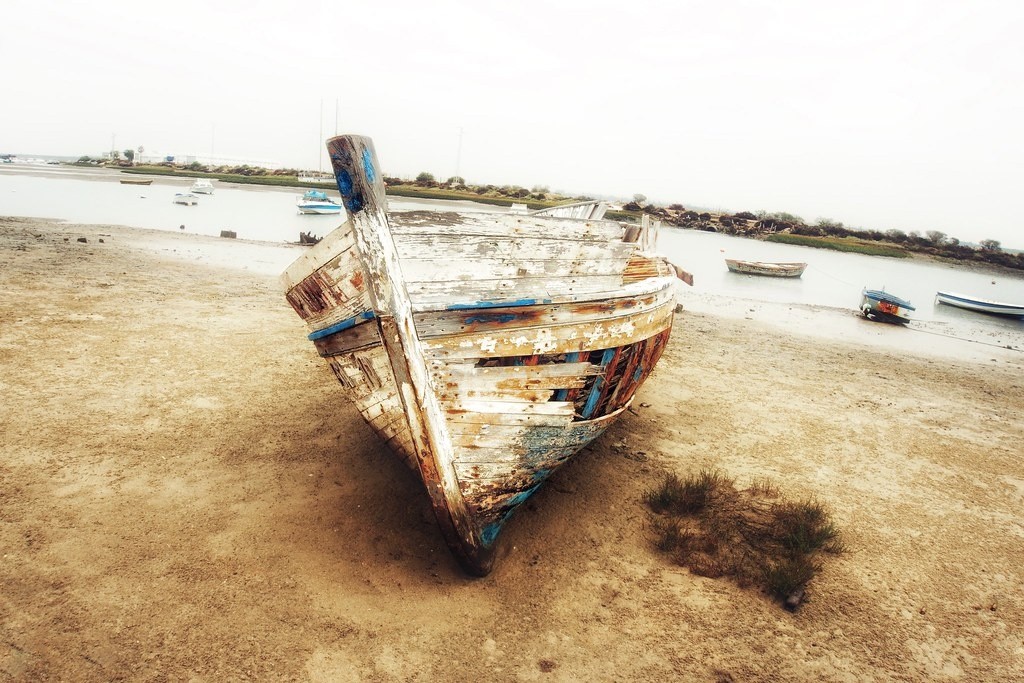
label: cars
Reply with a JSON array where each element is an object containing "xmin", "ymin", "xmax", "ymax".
[
  {"xmin": 4, "ymin": 160, "xmax": 13, "ymax": 163},
  {"xmin": 123, "ymin": 162, "xmax": 138, "ymax": 166},
  {"xmin": 54, "ymin": 161, "xmax": 59, "ymax": 165}
]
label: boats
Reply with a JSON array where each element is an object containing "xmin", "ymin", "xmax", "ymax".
[
  {"xmin": 279, "ymin": 135, "xmax": 694, "ymax": 578},
  {"xmin": 859, "ymin": 285, "xmax": 916, "ymax": 326},
  {"xmin": 296, "ymin": 197, "xmax": 342, "ymax": 215},
  {"xmin": 191, "ymin": 181, "xmax": 215, "ymax": 195},
  {"xmin": 937, "ymin": 290, "xmax": 1024, "ymax": 319},
  {"xmin": 120, "ymin": 179, "xmax": 153, "ymax": 185},
  {"xmin": 173, "ymin": 193, "xmax": 200, "ymax": 206},
  {"xmin": 725, "ymin": 258, "xmax": 808, "ymax": 278}
]
